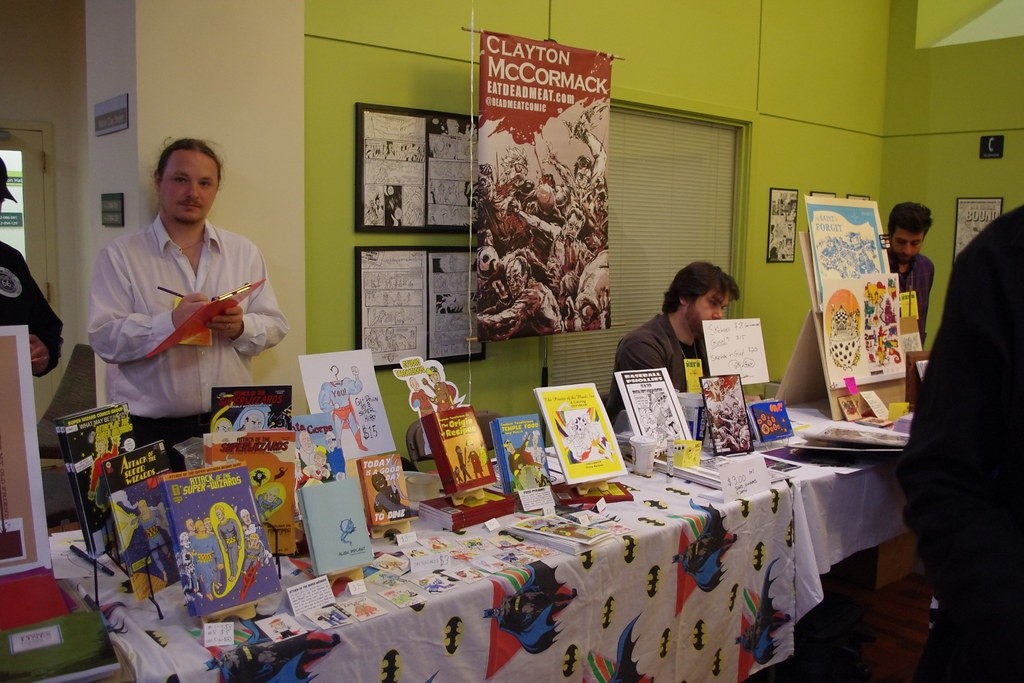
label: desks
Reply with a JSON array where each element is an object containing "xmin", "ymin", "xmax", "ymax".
[{"xmin": 56, "ymin": 405, "xmax": 904, "ymax": 681}]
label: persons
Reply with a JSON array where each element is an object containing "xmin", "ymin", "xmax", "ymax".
[
  {"xmin": 886, "ymin": 203, "xmax": 934, "ymax": 349},
  {"xmin": 894, "ymin": 206, "xmax": 1024, "ymax": 682},
  {"xmin": 605, "ymin": 260, "xmax": 740, "ymax": 425},
  {"xmin": 887, "ymin": 201, "xmax": 935, "ymax": 353},
  {"xmin": 92, "ymin": 140, "xmax": 289, "ymax": 471},
  {"xmin": 0, "ymin": 159, "xmax": 65, "ymax": 377}
]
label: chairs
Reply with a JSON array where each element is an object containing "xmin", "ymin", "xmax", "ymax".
[{"xmin": 405, "ymin": 411, "xmax": 505, "ymax": 475}]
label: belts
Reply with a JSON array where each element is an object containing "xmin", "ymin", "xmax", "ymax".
[{"xmin": 173, "ymin": 412, "xmax": 211, "ymax": 426}]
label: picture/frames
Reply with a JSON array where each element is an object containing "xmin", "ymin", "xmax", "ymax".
[
  {"xmin": 952, "ymin": 197, "xmax": 1003, "ymax": 266},
  {"xmin": 766, "ymin": 187, "xmax": 798, "ymax": 262},
  {"xmin": 354, "ymin": 102, "xmax": 477, "ymax": 232},
  {"xmin": 354, "ymin": 244, "xmax": 485, "ymax": 370}
]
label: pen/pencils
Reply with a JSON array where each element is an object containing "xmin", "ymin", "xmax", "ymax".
[
  {"xmin": 70, "ymin": 545, "xmax": 115, "ymax": 576},
  {"xmin": 774, "ymin": 592, "xmax": 876, "ymax": 683},
  {"xmin": 77, "ymin": 583, "xmax": 110, "ymax": 628},
  {"xmin": 157, "ymin": 286, "xmax": 184, "ymax": 298}
]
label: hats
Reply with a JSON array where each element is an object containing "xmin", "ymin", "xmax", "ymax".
[{"xmin": 0, "ymin": 159, "xmax": 18, "ymax": 204}]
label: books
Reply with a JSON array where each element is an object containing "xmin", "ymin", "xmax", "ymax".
[
  {"xmin": 746, "ymin": 397, "xmax": 794, "ymax": 443},
  {"xmin": 356, "ymin": 454, "xmax": 411, "ymax": 527},
  {"xmin": 699, "ymin": 374, "xmax": 755, "ymax": 457},
  {"xmin": 52, "ymin": 402, "xmax": 180, "ymax": 601},
  {"xmin": 614, "ymin": 367, "xmax": 692, "ymax": 452},
  {"xmin": 157, "ymin": 386, "xmax": 296, "ymax": 616},
  {"xmin": 298, "ymin": 480, "xmax": 374, "ymax": 576},
  {"xmin": 421, "ymin": 406, "xmax": 497, "ymax": 496},
  {"xmin": 489, "ymin": 412, "xmax": 552, "ymax": 495},
  {"xmin": 533, "ymin": 385, "xmax": 628, "ymax": 486},
  {"xmin": 291, "ymin": 412, "xmax": 349, "ymax": 516},
  {"xmin": 0, "ymin": 566, "xmax": 120, "ymax": 683}
]
